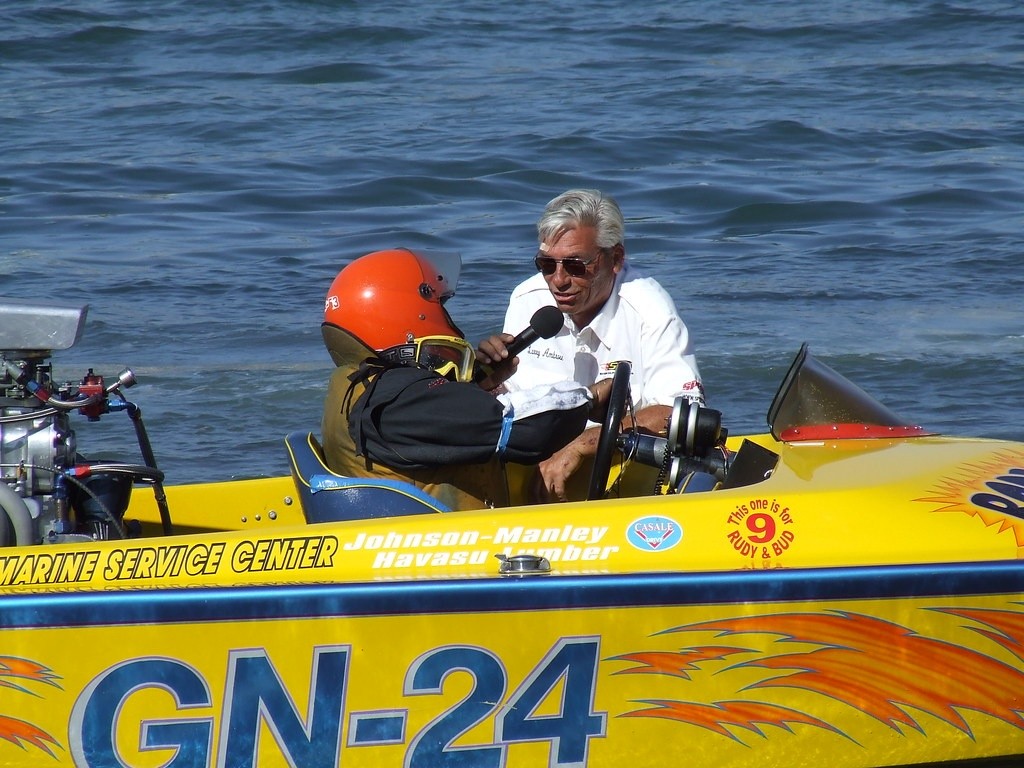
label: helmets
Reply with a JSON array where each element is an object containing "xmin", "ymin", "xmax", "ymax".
[{"xmin": 322, "ymin": 247, "xmax": 466, "ymax": 379}]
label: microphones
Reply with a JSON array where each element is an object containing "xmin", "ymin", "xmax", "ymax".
[{"xmin": 469, "ymin": 306, "xmax": 564, "ymax": 383}]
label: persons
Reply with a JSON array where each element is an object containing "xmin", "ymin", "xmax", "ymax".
[{"xmin": 322, "ymin": 187, "xmax": 707, "ymax": 512}]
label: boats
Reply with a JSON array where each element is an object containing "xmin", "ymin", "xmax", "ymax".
[{"xmin": 0, "ymin": 300, "xmax": 1024, "ymax": 768}]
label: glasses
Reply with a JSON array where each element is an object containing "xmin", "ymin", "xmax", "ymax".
[
  {"xmin": 413, "ymin": 335, "xmax": 477, "ymax": 382},
  {"xmin": 533, "ymin": 244, "xmax": 615, "ymax": 275}
]
